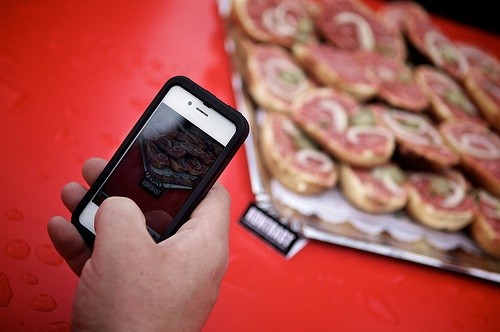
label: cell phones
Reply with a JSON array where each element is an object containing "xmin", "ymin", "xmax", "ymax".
[{"xmin": 70, "ymin": 75, "xmax": 250, "ymax": 251}]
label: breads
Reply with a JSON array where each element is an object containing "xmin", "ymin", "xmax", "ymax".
[
  {"xmin": 139, "ymin": 105, "xmax": 222, "ymax": 176},
  {"xmin": 236, "ymin": 0, "xmax": 499, "ymax": 259}
]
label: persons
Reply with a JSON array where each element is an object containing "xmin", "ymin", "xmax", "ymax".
[{"xmin": 46, "ymin": 157, "xmax": 229, "ymax": 332}]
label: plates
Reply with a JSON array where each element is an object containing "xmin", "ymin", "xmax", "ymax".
[{"xmin": 217, "ymin": 0, "xmax": 499, "ymax": 286}]
